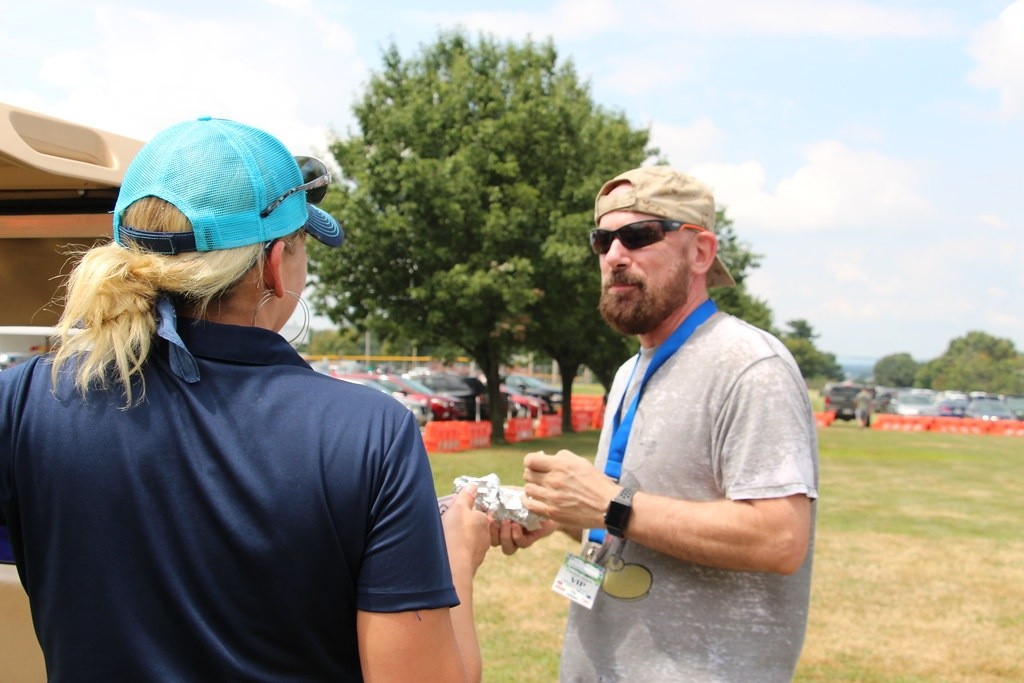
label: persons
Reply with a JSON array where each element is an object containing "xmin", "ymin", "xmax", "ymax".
[
  {"xmin": 0, "ymin": 118, "xmax": 492, "ymax": 683},
  {"xmin": 490, "ymin": 166, "xmax": 817, "ymax": 683},
  {"xmin": 854, "ymin": 388, "xmax": 871, "ymax": 427}
]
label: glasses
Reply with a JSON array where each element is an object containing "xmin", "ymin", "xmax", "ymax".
[
  {"xmin": 259, "ymin": 153, "xmax": 333, "ymax": 218},
  {"xmin": 590, "ymin": 215, "xmax": 709, "ymax": 257}
]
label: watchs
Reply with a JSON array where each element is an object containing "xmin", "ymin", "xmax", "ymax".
[{"xmin": 605, "ymin": 487, "xmax": 638, "ymax": 539}]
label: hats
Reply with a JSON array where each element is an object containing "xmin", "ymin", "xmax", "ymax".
[
  {"xmin": 112, "ymin": 115, "xmax": 343, "ymax": 255},
  {"xmin": 593, "ymin": 166, "xmax": 735, "ymax": 286}
]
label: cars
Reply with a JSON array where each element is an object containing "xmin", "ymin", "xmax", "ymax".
[
  {"xmin": 308, "ymin": 357, "xmax": 562, "ymax": 431},
  {"xmin": 819, "ymin": 381, "xmax": 1024, "ymax": 428}
]
